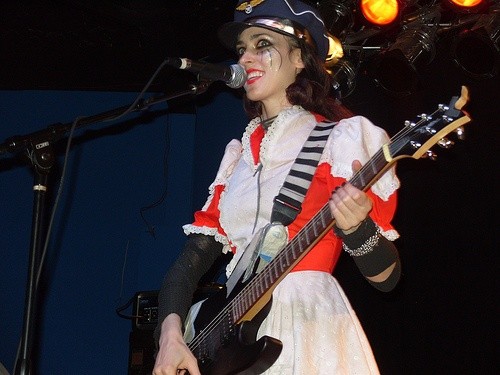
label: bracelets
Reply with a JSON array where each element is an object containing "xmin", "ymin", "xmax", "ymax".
[{"xmin": 342, "ymin": 222, "xmax": 382, "ymax": 257}]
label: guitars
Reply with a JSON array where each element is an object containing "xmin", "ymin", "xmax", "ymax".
[{"xmin": 177, "ymin": 86, "xmax": 473, "ymax": 375}]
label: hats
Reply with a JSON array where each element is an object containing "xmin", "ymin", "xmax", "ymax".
[{"xmin": 217, "ymin": 0, "xmax": 330, "ymax": 63}]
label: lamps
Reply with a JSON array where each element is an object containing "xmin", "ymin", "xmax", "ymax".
[{"xmin": 311, "ymin": 1, "xmax": 500, "ymax": 104}]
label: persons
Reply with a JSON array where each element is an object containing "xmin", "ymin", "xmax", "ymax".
[{"xmin": 151, "ymin": 0, "xmax": 401, "ymax": 375}]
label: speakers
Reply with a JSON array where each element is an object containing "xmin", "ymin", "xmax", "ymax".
[{"xmin": 128, "ymin": 330, "xmax": 160, "ymax": 375}]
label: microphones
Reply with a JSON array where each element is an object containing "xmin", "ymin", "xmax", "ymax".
[{"xmin": 165, "ymin": 57, "xmax": 247, "ymax": 89}]
